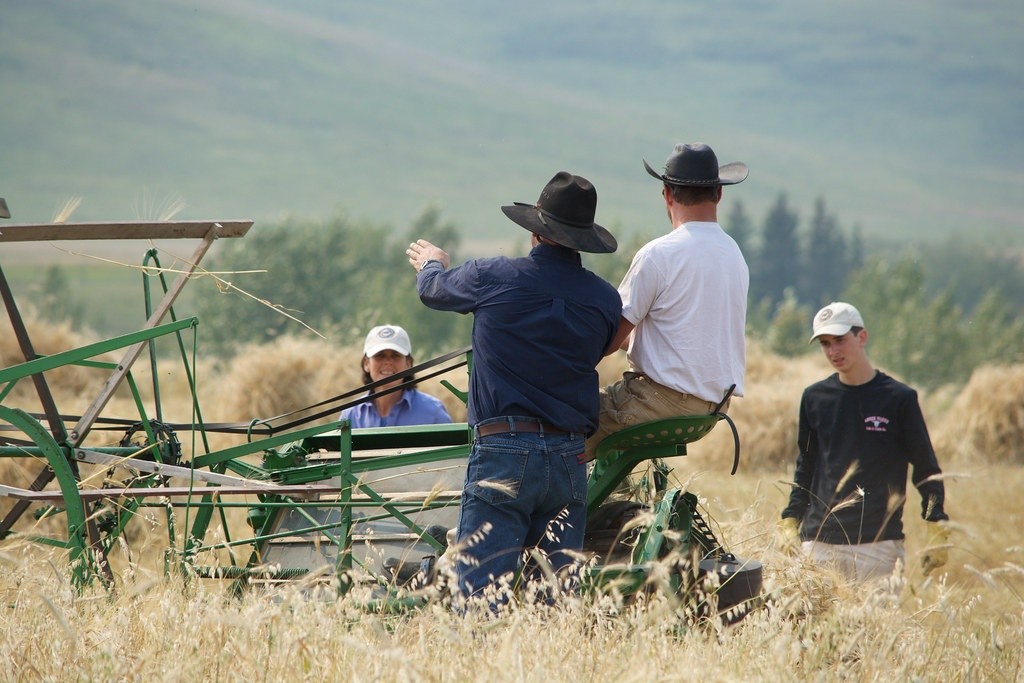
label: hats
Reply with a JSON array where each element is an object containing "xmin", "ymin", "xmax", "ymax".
[
  {"xmin": 503, "ymin": 169, "xmax": 619, "ymax": 256},
  {"xmin": 361, "ymin": 323, "xmax": 413, "ymax": 358},
  {"xmin": 641, "ymin": 140, "xmax": 751, "ymax": 188},
  {"xmin": 806, "ymin": 301, "xmax": 864, "ymax": 342}
]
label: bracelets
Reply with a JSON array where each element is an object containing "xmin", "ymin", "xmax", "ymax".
[{"xmin": 421, "ymin": 260, "xmax": 445, "ymax": 271}]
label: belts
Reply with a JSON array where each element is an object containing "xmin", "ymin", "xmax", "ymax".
[{"xmin": 480, "ymin": 420, "xmax": 574, "ymax": 435}]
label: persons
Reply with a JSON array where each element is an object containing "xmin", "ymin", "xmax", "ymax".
[
  {"xmin": 779, "ymin": 302, "xmax": 949, "ymax": 611},
  {"xmin": 406, "ymin": 172, "xmax": 622, "ymax": 614},
  {"xmin": 339, "ymin": 325, "xmax": 452, "ymax": 428},
  {"xmin": 580, "ymin": 142, "xmax": 751, "ymax": 464}
]
